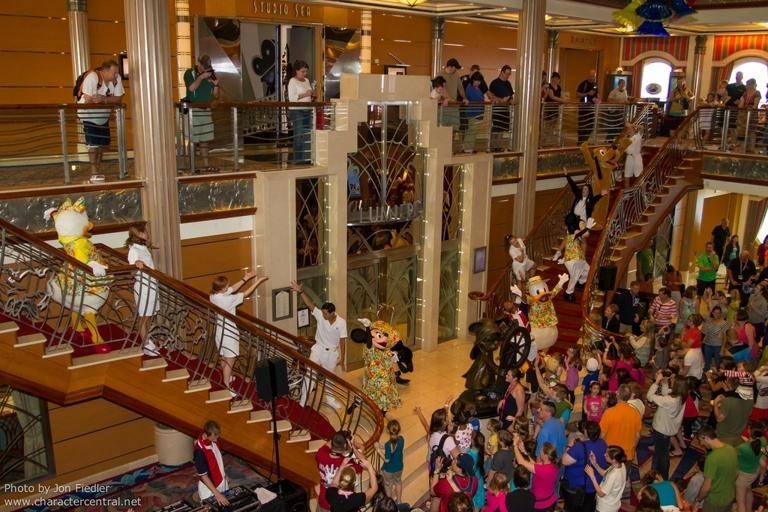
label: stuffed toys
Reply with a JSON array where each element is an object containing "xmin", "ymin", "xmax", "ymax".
[{"xmin": 46, "ymin": 196, "xmax": 115, "ymax": 345}]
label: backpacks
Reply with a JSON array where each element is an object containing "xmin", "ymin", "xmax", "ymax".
[{"xmin": 73, "ymin": 67, "xmax": 117, "ymax": 101}]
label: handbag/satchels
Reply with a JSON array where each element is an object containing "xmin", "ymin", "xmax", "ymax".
[{"xmin": 560, "ymin": 480, "xmax": 586, "ymax": 508}]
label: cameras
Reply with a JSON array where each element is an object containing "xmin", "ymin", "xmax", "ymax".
[
  {"xmin": 207, "ymin": 70, "xmax": 216, "ymax": 81},
  {"xmin": 342, "ymin": 430, "xmax": 352, "ymax": 442},
  {"xmin": 663, "ymin": 369, "xmax": 671, "ymax": 377}
]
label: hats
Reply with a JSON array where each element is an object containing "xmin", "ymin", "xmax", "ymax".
[
  {"xmin": 197, "ymin": 54, "xmax": 212, "ymax": 69},
  {"xmin": 544, "ymin": 355, "xmax": 558, "ymax": 372},
  {"xmin": 587, "ymin": 357, "xmax": 598, "ymax": 372},
  {"xmin": 459, "ymin": 453, "xmax": 476, "ymax": 478},
  {"xmin": 447, "ymin": 59, "xmax": 462, "ymax": 70}
]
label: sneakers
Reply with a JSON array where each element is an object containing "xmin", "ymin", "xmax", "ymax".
[
  {"xmin": 552, "ymin": 250, "xmax": 562, "ymax": 262},
  {"xmin": 143, "ymin": 339, "xmax": 160, "ymax": 357},
  {"xmin": 326, "ymin": 401, "xmax": 342, "ymax": 408}
]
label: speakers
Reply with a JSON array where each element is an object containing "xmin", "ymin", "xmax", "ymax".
[
  {"xmin": 598, "ymin": 266, "xmax": 617, "ymax": 291},
  {"xmin": 254, "ymin": 355, "xmax": 289, "ymax": 400}
]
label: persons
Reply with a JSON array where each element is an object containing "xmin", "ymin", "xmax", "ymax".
[{"xmin": 71, "ymin": 55, "xmax": 768, "ymax": 512}]
